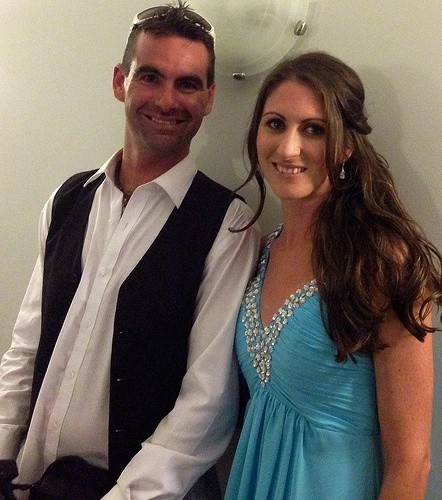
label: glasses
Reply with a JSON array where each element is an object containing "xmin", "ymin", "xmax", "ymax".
[{"xmin": 122, "ymin": 5, "xmax": 217, "ymax": 62}]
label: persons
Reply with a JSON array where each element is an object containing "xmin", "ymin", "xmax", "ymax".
[
  {"xmin": 226, "ymin": 52, "xmax": 442, "ymax": 500},
  {"xmin": 1, "ymin": 3, "xmax": 264, "ymax": 500}
]
